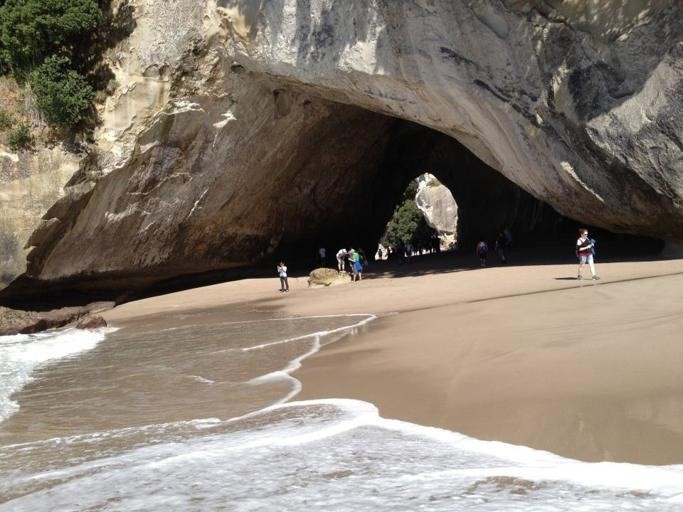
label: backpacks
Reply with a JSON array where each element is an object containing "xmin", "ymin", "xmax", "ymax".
[{"xmin": 478, "ymin": 242, "xmax": 488, "ymax": 256}]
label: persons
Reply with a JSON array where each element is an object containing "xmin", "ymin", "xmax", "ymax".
[
  {"xmin": 346, "ymin": 246, "xmax": 368, "ymax": 282},
  {"xmin": 474, "ymin": 228, "xmax": 516, "ymax": 270},
  {"xmin": 574, "ymin": 226, "xmax": 600, "ymax": 280},
  {"xmin": 377, "ymin": 234, "xmax": 465, "ymax": 260},
  {"xmin": 334, "ymin": 248, "xmax": 347, "ymax": 273},
  {"xmin": 275, "ymin": 261, "xmax": 289, "ymax": 291},
  {"xmin": 318, "ymin": 244, "xmax": 326, "ymax": 267}
]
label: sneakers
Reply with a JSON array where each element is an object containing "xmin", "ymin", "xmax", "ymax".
[
  {"xmin": 577, "ymin": 274, "xmax": 584, "ymax": 281},
  {"xmin": 281, "ymin": 288, "xmax": 289, "ymax": 292},
  {"xmin": 339, "ymin": 270, "xmax": 346, "ymax": 273},
  {"xmin": 590, "ymin": 275, "xmax": 600, "ymax": 282}
]
